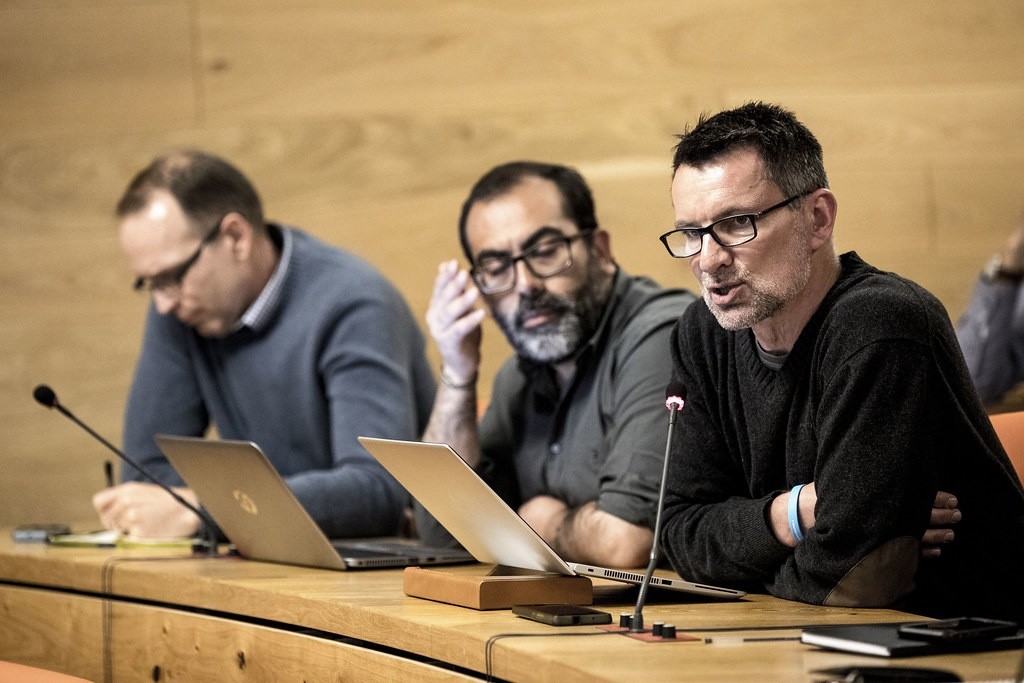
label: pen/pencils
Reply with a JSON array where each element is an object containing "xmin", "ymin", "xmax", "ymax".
[{"xmin": 104, "ymin": 460, "xmax": 115, "ymax": 487}]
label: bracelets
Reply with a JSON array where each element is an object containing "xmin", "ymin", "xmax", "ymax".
[
  {"xmin": 787, "ymin": 483, "xmax": 805, "ymax": 544},
  {"xmin": 439, "ymin": 363, "xmax": 480, "ymax": 389}
]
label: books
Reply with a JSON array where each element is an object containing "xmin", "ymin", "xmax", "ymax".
[
  {"xmin": 801, "ymin": 617, "xmax": 1023, "ymax": 658},
  {"xmin": 47, "ymin": 527, "xmax": 121, "ymax": 549}
]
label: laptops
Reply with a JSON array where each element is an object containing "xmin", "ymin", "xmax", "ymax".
[
  {"xmin": 152, "ymin": 433, "xmax": 478, "ymax": 570},
  {"xmin": 356, "ymin": 436, "xmax": 746, "ymax": 599}
]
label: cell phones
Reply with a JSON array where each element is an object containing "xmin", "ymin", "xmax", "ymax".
[
  {"xmin": 898, "ymin": 614, "xmax": 1018, "ymax": 645},
  {"xmin": 511, "ymin": 603, "xmax": 612, "ymax": 626}
]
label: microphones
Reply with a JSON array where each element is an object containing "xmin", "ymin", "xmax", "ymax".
[
  {"xmin": 33, "ymin": 384, "xmax": 218, "ymax": 557},
  {"xmin": 630, "ymin": 382, "xmax": 690, "ymax": 632}
]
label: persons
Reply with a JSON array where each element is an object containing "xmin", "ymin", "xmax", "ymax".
[
  {"xmin": 92, "ymin": 149, "xmax": 440, "ymax": 539},
  {"xmin": 414, "ymin": 158, "xmax": 703, "ymax": 571},
  {"xmin": 948, "ymin": 226, "xmax": 1024, "ymax": 407},
  {"xmin": 655, "ymin": 101, "xmax": 1024, "ymax": 623}
]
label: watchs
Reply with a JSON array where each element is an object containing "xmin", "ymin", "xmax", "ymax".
[{"xmin": 985, "ymin": 256, "xmax": 1023, "ymax": 287}]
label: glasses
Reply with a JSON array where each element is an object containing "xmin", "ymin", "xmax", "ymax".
[
  {"xmin": 469, "ymin": 228, "xmax": 598, "ymax": 296},
  {"xmin": 133, "ymin": 217, "xmax": 224, "ymax": 297},
  {"xmin": 659, "ymin": 187, "xmax": 822, "ymax": 259}
]
